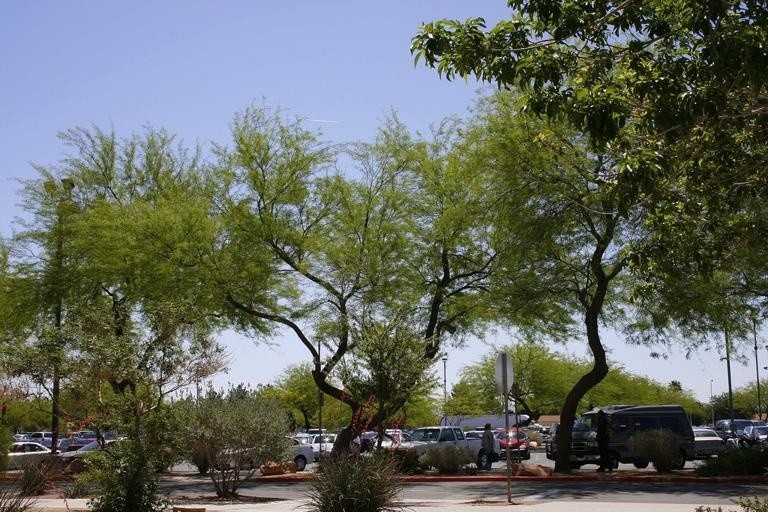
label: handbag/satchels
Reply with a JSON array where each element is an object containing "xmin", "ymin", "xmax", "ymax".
[{"xmin": 486, "ymin": 452, "xmax": 498, "ymax": 462}]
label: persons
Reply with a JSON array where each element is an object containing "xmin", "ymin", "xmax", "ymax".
[
  {"xmin": 592, "ymin": 410, "xmax": 614, "ymax": 473},
  {"xmin": 481, "ymin": 423, "xmax": 496, "ymax": 472}
]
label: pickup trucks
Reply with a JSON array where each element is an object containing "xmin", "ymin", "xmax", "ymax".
[{"xmin": 384, "ymin": 425, "xmax": 501, "ymax": 472}]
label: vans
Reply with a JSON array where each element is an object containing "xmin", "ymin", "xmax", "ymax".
[{"xmin": 569, "ymin": 403, "xmax": 697, "ymax": 469}]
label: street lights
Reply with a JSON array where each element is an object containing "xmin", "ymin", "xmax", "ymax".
[
  {"xmin": 313, "ymin": 336, "xmax": 325, "ymax": 459},
  {"xmin": 709, "ymin": 379, "xmax": 716, "ymax": 428},
  {"xmin": 441, "ymin": 353, "xmax": 449, "ymax": 424},
  {"xmin": 44, "ymin": 174, "xmax": 75, "ymax": 456}
]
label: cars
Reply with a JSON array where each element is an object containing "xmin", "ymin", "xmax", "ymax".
[
  {"xmin": 292, "ymin": 425, "xmax": 413, "ymax": 461},
  {"xmin": 465, "ymin": 420, "xmax": 580, "ymax": 464},
  {"xmin": 690, "ymin": 418, "xmax": 767, "ymax": 461},
  {"xmin": 1, "ymin": 429, "xmax": 172, "ymax": 478},
  {"xmin": 216, "ymin": 435, "xmax": 314, "ymax": 471}
]
